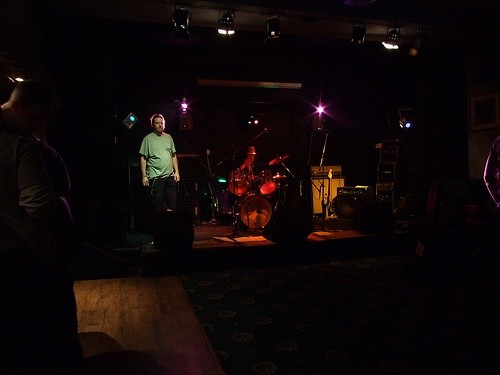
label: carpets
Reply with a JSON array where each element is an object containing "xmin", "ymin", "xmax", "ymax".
[{"xmin": 180, "ymin": 256, "xmax": 500, "ymax": 375}]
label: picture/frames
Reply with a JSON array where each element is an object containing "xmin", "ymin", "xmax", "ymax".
[{"xmin": 469, "ymin": 92, "xmax": 500, "ymax": 132}]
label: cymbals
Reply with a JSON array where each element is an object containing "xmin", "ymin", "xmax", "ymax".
[
  {"xmin": 273, "ymin": 176, "xmax": 287, "ymax": 179},
  {"xmin": 269, "ymin": 154, "xmax": 289, "ymax": 166}
]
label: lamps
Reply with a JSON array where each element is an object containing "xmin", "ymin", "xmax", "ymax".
[
  {"xmin": 349, "ymin": 22, "xmax": 366, "ymax": 47},
  {"xmin": 409, "ymin": 28, "xmax": 425, "ymax": 55},
  {"xmin": 217, "ymin": 10, "xmax": 237, "ymax": 34},
  {"xmin": 167, "ymin": 4, "xmax": 192, "ymax": 34},
  {"xmin": 383, "ymin": 24, "xmax": 403, "ymax": 50},
  {"xmin": 266, "ymin": 17, "xmax": 281, "ymax": 41}
]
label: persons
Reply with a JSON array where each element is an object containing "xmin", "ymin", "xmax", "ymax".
[
  {"xmin": 139, "ymin": 114, "xmax": 180, "ymax": 245},
  {"xmin": 262, "ymin": 184, "xmax": 315, "ymax": 265},
  {"xmin": 0, "ymin": 51, "xmax": 83, "ymax": 375}
]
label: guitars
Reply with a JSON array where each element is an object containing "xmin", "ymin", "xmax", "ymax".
[{"xmin": 327, "ymin": 168, "xmax": 335, "ymax": 213}]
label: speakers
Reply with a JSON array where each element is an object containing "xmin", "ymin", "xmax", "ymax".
[
  {"xmin": 336, "ymin": 186, "xmax": 373, "ymax": 220},
  {"xmin": 152, "ymin": 208, "xmax": 195, "ymax": 249},
  {"xmin": 263, "ymin": 197, "xmax": 314, "ymax": 243},
  {"xmin": 311, "ymin": 176, "xmax": 345, "ymax": 215},
  {"xmin": 350, "ymin": 200, "xmax": 397, "ymax": 235}
]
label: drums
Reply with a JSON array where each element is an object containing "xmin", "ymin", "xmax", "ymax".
[
  {"xmin": 227, "ymin": 168, "xmax": 251, "ymax": 197},
  {"xmin": 217, "ymin": 189, "xmax": 238, "ymax": 214},
  {"xmin": 255, "ymin": 170, "xmax": 277, "ymax": 195},
  {"xmin": 238, "ymin": 192, "xmax": 273, "ymax": 229}
]
